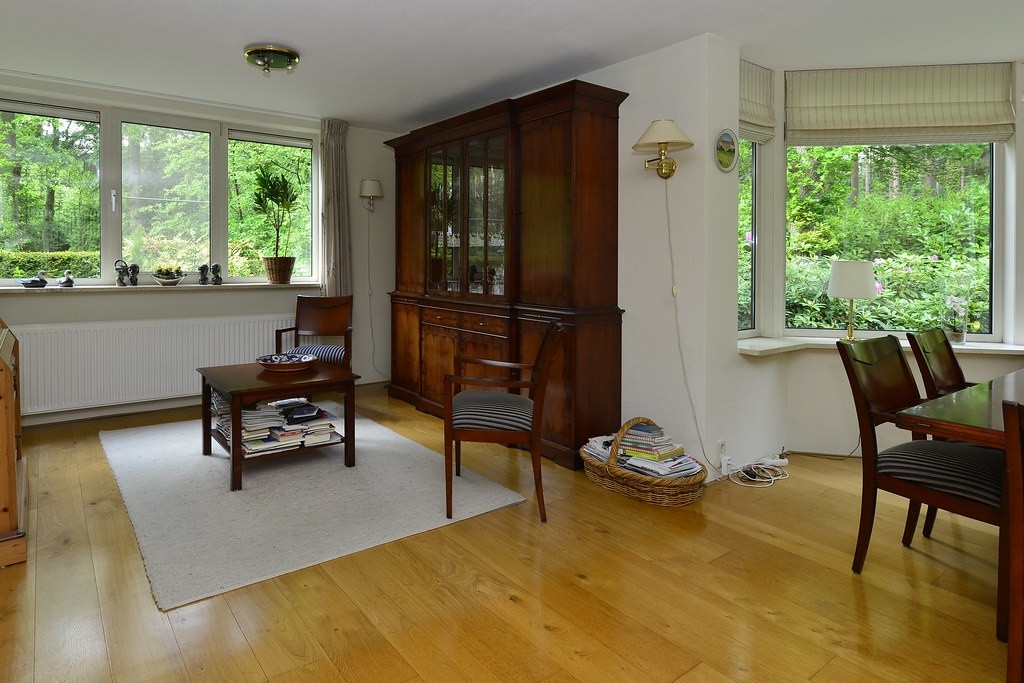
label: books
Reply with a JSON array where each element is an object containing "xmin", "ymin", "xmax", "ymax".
[
  {"xmin": 619, "ymin": 424, "xmax": 684, "ymax": 461},
  {"xmin": 211, "ymin": 387, "xmax": 335, "ymax": 457}
]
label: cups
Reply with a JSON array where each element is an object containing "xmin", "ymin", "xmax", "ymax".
[{"xmin": 438, "ymin": 169, "xmax": 504, "ymax": 295}]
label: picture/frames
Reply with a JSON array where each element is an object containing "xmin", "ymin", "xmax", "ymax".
[{"xmin": 716, "ymin": 129, "xmax": 739, "ymax": 172}]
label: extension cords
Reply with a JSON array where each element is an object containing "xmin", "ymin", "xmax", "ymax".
[{"xmin": 760, "ymin": 457, "xmax": 788, "ymax": 466}]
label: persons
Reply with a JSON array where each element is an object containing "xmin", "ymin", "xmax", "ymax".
[
  {"xmin": 211, "ymin": 264, "xmax": 223, "ymax": 285},
  {"xmin": 129, "ymin": 263, "xmax": 139, "ymax": 282},
  {"xmin": 198, "ymin": 264, "xmax": 209, "ymax": 285}
]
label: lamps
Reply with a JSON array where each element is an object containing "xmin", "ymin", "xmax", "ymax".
[
  {"xmin": 828, "ymin": 260, "xmax": 876, "ymax": 341},
  {"xmin": 360, "ymin": 180, "xmax": 383, "ymax": 211},
  {"xmin": 632, "ymin": 120, "xmax": 693, "ymax": 179},
  {"xmin": 243, "ymin": 45, "xmax": 299, "ymax": 75}
]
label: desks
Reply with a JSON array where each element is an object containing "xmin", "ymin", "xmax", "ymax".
[
  {"xmin": 192, "ymin": 363, "xmax": 362, "ymax": 494},
  {"xmin": 895, "ymin": 368, "xmax": 1024, "ymax": 642}
]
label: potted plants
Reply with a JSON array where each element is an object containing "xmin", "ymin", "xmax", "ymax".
[{"xmin": 253, "ymin": 164, "xmax": 299, "ymax": 284}]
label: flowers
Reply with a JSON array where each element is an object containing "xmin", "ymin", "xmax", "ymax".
[
  {"xmin": 156, "ymin": 265, "xmax": 181, "ymax": 276},
  {"xmin": 942, "ymin": 293, "xmax": 969, "ymax": 332}
]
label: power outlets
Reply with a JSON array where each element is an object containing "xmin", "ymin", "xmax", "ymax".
[{"xmin": 721, "ymin": 457, "xmax": 732, "ymax": 475}]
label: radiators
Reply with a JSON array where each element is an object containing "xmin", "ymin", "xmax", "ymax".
[{"xmin": 6, "ymin": 312, "xmax": 323, "ymax": 416}]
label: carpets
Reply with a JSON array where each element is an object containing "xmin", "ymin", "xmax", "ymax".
[{"xmin": 98, "ymin": 399, "xmax": 523, "ymax": 612}]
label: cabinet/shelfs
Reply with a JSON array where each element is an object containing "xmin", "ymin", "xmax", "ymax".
[{"xmin": 384, "ymin": 78, "xmax": 630, "ymax": 471}]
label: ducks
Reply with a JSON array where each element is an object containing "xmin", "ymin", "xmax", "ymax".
[
  {"xmin": 17, "ymin": 270, "xmax": 48, "ymax": 288},
  {"xmin": 57, "ymin": 270, "xmax": 75, "ymax": 287}
]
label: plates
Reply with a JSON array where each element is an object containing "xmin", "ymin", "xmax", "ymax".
[{"xmin": 255, "ymin": 354, "xmax": 318, "ymax": 372}]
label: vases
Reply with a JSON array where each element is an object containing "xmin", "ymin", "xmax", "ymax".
[
  {"xmin": 944, "ymin": 328, "xmax": 966, "ymax": 344},
  {"xmin": 152, "ymin": 273, "xmax": 187, "ymax": 286}
]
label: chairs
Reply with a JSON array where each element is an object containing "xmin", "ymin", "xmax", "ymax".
[
  {"xmin": 443, "ymin": 319, "xmax": 564, "ymax": 522},
  {"xmin": 276, "ymin": 295, "xmax": 353, "ymax": 403},
  {"xmin": 1000, "ymin": 399, "xmax": 1024, "ymax": 683},
  {"xmin": 834, "ymin": 334, "xmax": 1010, "ymax": 642},
  {"xmin": 906, "ymin": 326, "xmax": 981, "ymax": 539}
]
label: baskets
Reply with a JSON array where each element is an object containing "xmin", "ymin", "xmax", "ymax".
[{"xmin": 580, "ymin": 417, "xmax": 708, "ymax": 507}]
